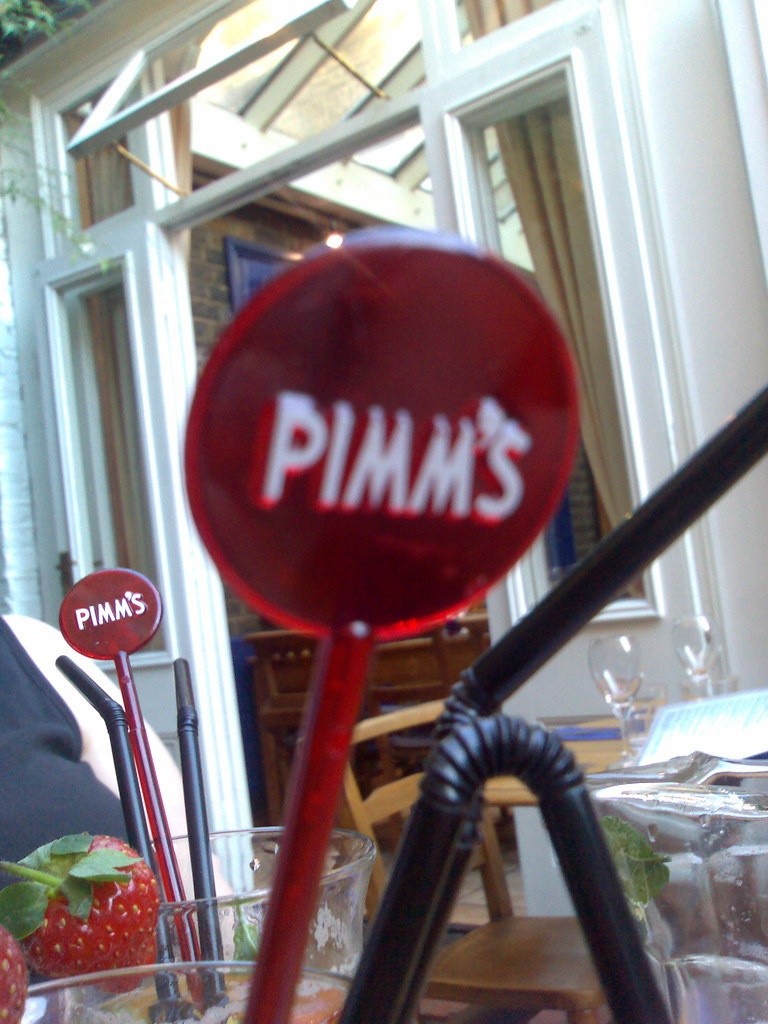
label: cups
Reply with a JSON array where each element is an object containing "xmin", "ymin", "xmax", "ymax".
[
  {"xmin": 18, "ymin": 961, "xmax": 420, "ymax": 1024},
  {"xmin": 589, "ymin": 782, "xmax": 768, "ymax": 1024},
  {"xmin": 48, "ymin": 826, "xmax": 376, "ymax": 1024},
  {"xmin": 660, "ymin": 613, "xmax": 737, "ymax": 699}
]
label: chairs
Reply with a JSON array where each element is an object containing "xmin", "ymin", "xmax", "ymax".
[{"xmin": 335, "ymin": 697, "xmax": 615, "ymax": 1024}]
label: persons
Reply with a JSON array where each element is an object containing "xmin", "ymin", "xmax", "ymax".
[
  {"xmin": 0, "ymin": 613, "xmax": 236, "ymax": 985},
  {"xmin": 231, "ymin": 614, "xmax": 280, "ymax": 808}
]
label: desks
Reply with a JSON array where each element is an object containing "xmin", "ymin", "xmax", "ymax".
[
  {"xmin": 244, "ymin": 617, "xmax": 492, "ymax": 827},
  {"xmin": 474, "ymin": 708, "xmax": 656, "ymax": 807}
]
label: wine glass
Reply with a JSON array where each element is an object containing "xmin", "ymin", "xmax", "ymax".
[{"xmin": 586, "ymin": 635, "xmax": 643, "ymax": 771}]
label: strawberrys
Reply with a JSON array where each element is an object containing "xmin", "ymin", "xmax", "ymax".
[
  {"xmin": 0, "ymin": 921, "xmax": 33, "ymax": 1024},
  {"xmin": 13, "ymin": 835, "xmax": 162, "ymax": 992}
]
